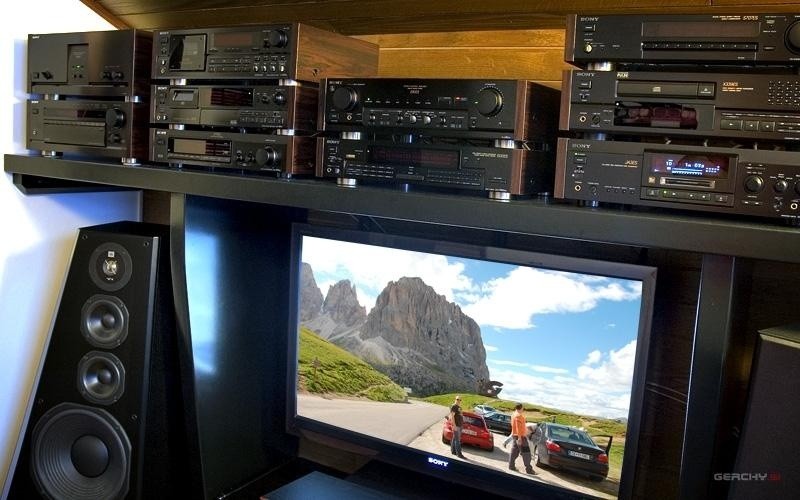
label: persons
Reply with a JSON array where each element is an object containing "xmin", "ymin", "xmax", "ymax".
[
  {"xmin": 502, "ymin": 422, "xmax": 541, "ymax": 456},
  {"xmin": 449, "ymin": 395, "xmax": 467, "ymax": 460},
  {"xmin": 509, "ymin": 403, "xmax": 538, "ymax": 476}
]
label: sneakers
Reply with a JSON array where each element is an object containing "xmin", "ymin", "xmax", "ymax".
[
  {"xmin": 452, "ymin": 451, "xmax": 468, "ymax": 460},
  {"xmin": 509, "ymin": 467, "xmax": 539, "ymax": 475},
  {"xmin": 502, "ymin": 442, "xmax": 522, "ymax": 455}
]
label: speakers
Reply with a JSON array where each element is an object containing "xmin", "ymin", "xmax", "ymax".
[{"xmin": 6, "ymin": 219, "xmax": 200, "ymax": 500}]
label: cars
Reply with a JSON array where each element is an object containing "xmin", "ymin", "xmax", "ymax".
[
  {"xmin": 441, "ymin": 404, "xmax": 516, "ymax": 449},
  {"xmin": 530, "ymin": 420, "xmax": 613, "ymax": 484}
]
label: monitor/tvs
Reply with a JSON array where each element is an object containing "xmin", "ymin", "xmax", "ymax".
[{"xmin": 284, "ymin": 223, "xmax": 659, "ymax": 499}]
